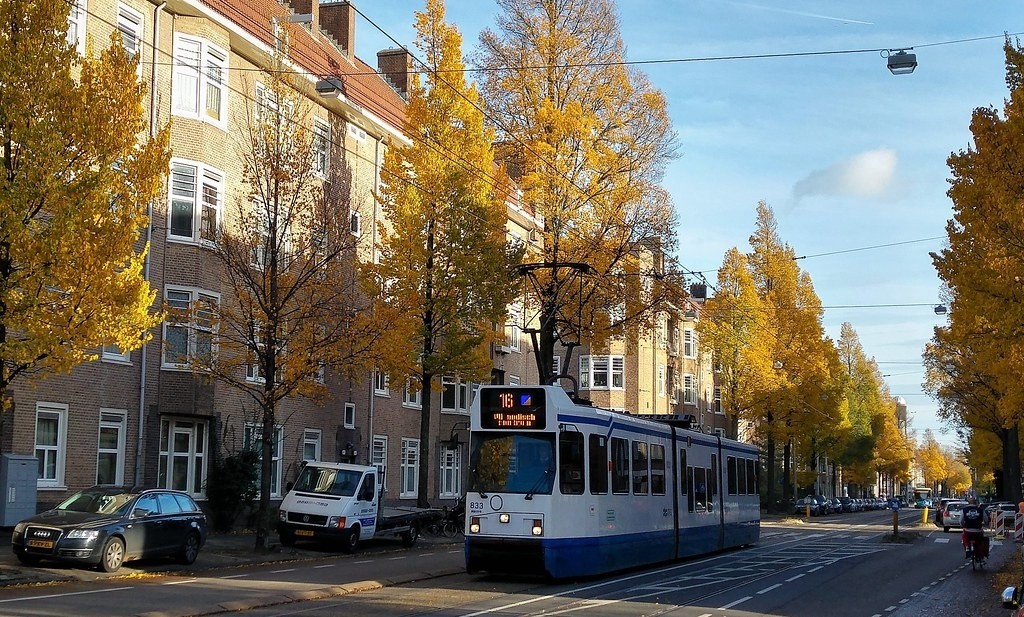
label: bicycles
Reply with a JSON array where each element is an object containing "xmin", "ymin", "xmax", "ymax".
[
  {"xmin": 962, "ymin": 520, "xmax": 989, "ymax": 571},
  {"xmin": 441, "ymin": 495, "xmax": 466, "ymax": 538}
]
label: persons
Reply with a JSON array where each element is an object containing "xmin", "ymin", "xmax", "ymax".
[{"xmin": 959, "ymin": 498, "xmax": 989, "ymax": 558}]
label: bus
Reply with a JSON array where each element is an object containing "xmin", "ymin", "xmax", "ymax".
[
  {"xmin": 446, "ymin": 262, "xmax": 761, "ymax": 583},
  {"xmin": 913, "ymin": 487, "xmax": 933, "ymax": 509}
]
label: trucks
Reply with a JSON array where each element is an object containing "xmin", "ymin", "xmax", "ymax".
[{"xmin": 277, "ymin": 461, "xmax": 433, "ymax": 554}]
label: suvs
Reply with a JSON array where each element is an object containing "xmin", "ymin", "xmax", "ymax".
[
  {"xmin": 11, "ymin": 483, "xmax": 208, "ymax": 574},
  {"xmin": 934, "ymin": 498, "xmax": 966, "ymax": 526}
]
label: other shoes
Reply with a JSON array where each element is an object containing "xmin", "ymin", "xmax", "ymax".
[
  {"xmin": 965, "ymin": 547, "xmax": 970, "ymax": 552},
  {"xmin": 981, "ymin": 560, "xmax": 987, "ymax": 566}
]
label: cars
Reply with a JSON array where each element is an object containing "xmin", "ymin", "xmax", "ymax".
[
  {"xmin": 1001, "ymin": 583, "xmax": 1024, "ymax": 617},
  {"xmin": 793, "ymin": 493, "xmax": 909, "ymax": 516},
  {"xmin": 940, "ymin": 502, "xmax": 970, "ymax": 532},
  {"xmin": 976, "ymin": 499, "xmax": 1016, "ymax": 528},
  {"xmin": 932, "ymin": 496, "xmax": 943, "ymax": 506}
]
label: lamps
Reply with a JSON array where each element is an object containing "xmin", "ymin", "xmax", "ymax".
[
  {"xmin": 774, "ymin": 361, "xmax": 783, "ymax": 369},
  {"xmin": 887, "ymin": 48, "xmax": 918, "ymax": 75},
  {"xmin": 935, "ymin": 304, "xmax": 947, "ymax": 315},
  {"xmin": 821, "ymin": 392, "xmax": 828, "ymax": 400},
  {"xmin": 315, "ymin": 74, "xmax": 342, "ymax": 98}
]
